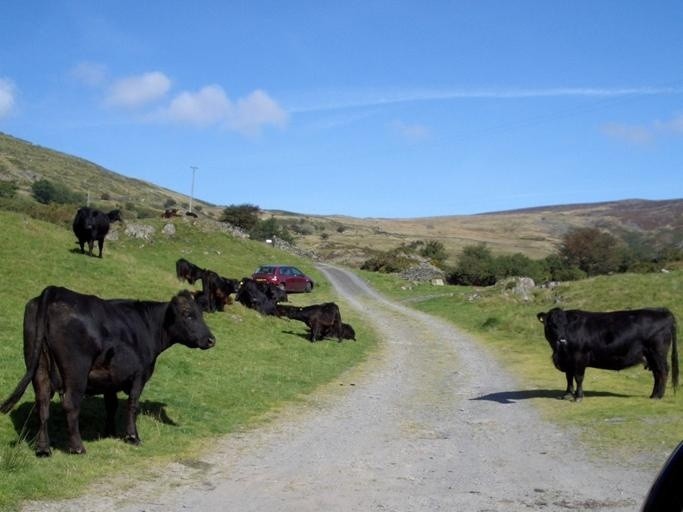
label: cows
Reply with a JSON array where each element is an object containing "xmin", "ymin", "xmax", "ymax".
[
  {"xmin": 175, "ymin": 258, "xmax": 356, "ymax": 343},
  {"xmin": 536, "ymin": 307, "xmax": 679, "ymax": 403},
  {"xmin": 162, "ymin": 209, "xmax": 198, "ymax": 219},
  {"xmin": 105, "ymin": 208, "xmax": 123, "ymax": 223},
  {"xmin": 72, "ymin": 206, "xmax": 110, "ymax": 258},
  {"xmin": 0, "ymin": 285, "xmax": 216, "ymax": 458}
]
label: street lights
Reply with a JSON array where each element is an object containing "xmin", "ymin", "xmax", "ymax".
[{"xmin": 186, "ymin": 165, "xmax": 199, "ymax": 212}]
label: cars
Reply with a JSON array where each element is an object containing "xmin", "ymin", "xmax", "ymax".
[{"xmin": 250, "ymin": 264, "xmax": 315, "ymax": 293}]
